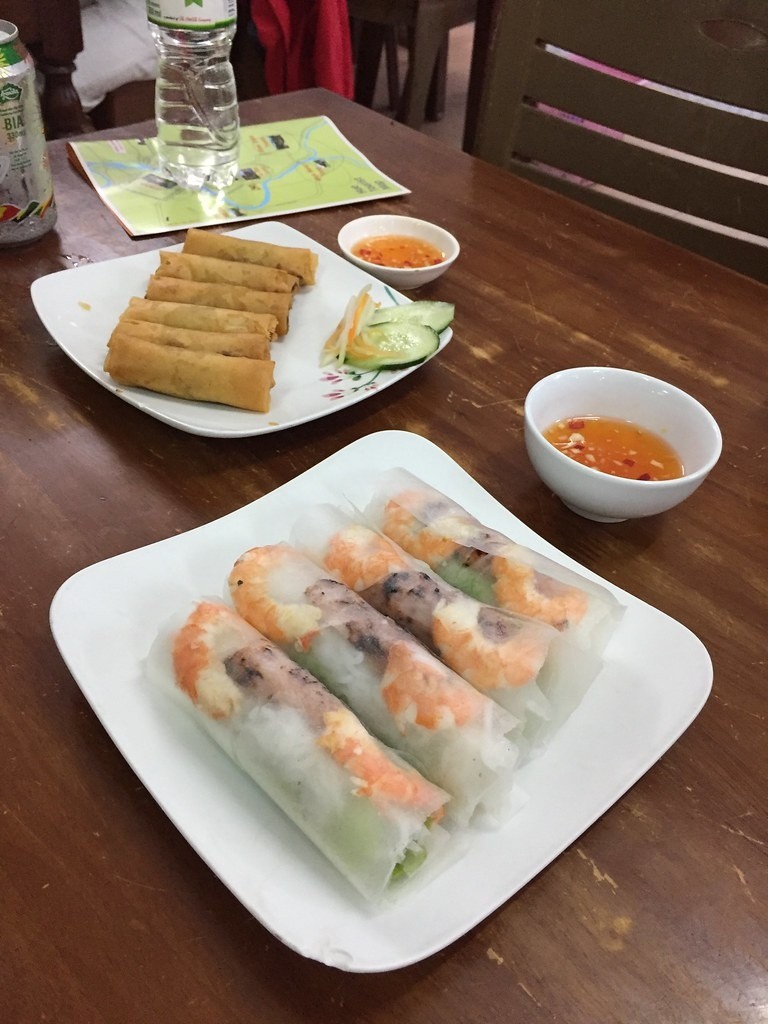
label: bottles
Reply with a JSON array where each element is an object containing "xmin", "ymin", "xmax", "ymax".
[{"xmin": 145, "ymin": 0, "xmax": 241, "ymax": 188}]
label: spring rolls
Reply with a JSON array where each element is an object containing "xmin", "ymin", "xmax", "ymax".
[
  {"xmin": 146, "ymin": 470, "xmax": 623, "ymax": 904},
  {"xmin": 104, "ymin": 226, "xmax": 319, "ymax": 412}
]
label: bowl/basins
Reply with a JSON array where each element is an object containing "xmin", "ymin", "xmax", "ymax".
[
  {"xmin": 523, "ymin": 367, "xmax": 724, "ymax": 522},
  {"xmin": 337, "ymin": 214, "xmax": 462, "ymax": 289}
]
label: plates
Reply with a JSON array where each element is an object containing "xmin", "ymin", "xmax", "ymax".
[
  {"xmin": 47, "ymin": 429, "xmax": 716, "ymax": 975},
  {"xmin": 32, "ymin": 221, "xmax": 455, "ymax": 440}
]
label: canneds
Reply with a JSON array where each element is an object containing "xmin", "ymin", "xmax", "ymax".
[{"xmin": 0, "ymin": 19, "xmax": 57, "ymax": 247}]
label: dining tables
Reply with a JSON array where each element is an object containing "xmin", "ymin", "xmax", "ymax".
[{"xmin": 0, "ymin": 86, "xmax": 768, "ymax": 1024}]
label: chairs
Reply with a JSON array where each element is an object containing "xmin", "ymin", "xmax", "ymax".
[{"xmin": 336, "ymin": 0, "xmax": 768, "ymax": 283}]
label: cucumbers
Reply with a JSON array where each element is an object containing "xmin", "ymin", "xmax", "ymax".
[
  {"xmin": 368, "ymin": 299, "xmax": 455, "ymax": 335},
  {"xmin": 339, "ymin": 320, "xmax": 440, "ymax": 368}
]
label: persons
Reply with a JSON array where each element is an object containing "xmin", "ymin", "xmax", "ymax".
[{"xmin": 24, "ymin": 0, "xmax": 161, "ymax": 136}]
